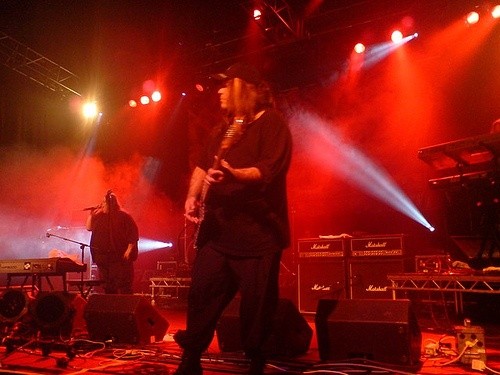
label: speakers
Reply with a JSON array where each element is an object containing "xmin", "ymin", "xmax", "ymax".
[
  {"xmin": 214, "ymin": 296, "xmax": 313, "ymax": 361},
  {"xmin": 32, "ymin": 290, "xmax": 86, "ymax": 339},
  {"xmin": 298, "ymin": 257, "xmax": 347, "ymax": 313},
  {"xmin": 349, "ymin": 257, "xmax": 412, "ymax": 300},
  {"xmin": 83, "ymin": 292, "xmax": 169, "ymax": 345},
  {"xmin": 313, "ymin": 298, "xmax": 421, "ymax": 371}
]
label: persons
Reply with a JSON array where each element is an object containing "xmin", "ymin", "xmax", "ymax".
[
  {"xmin": 171, "ymin": 64, "xmax": 293, "ymax": 375},
  {"xmin": 86, "ymin": 190, "xmax": 139, "ymax": 294}
]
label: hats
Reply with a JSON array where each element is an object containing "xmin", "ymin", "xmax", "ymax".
[{"xmin": 211, "ymin": 63, "xmax": 264, "ymax": 85}]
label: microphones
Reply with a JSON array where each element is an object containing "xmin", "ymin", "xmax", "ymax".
[{"xmin": 81, "ymin": 206, "xmax": 104, "ymax": 211}]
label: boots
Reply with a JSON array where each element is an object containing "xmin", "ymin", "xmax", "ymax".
[
  {"xmin": 243, "ymin": 357, "xmax": 267, "ymax": 375},
  {"xmin": 172, "ymin": 348, "xmax": 203, "ymax": 375}
]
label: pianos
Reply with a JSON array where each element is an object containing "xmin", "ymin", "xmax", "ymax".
[
  {"xmin": 0, "ymin": 258, "xmax": 89, "ymax": 299},
  {"xmin": 417, "ymin": 136, "xmax": 500, "ymax": 270}
]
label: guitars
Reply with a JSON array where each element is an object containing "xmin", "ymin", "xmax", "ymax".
[{"xmin": 192, "ymin": 112, "xmax": 246, "ymax": 251}]
